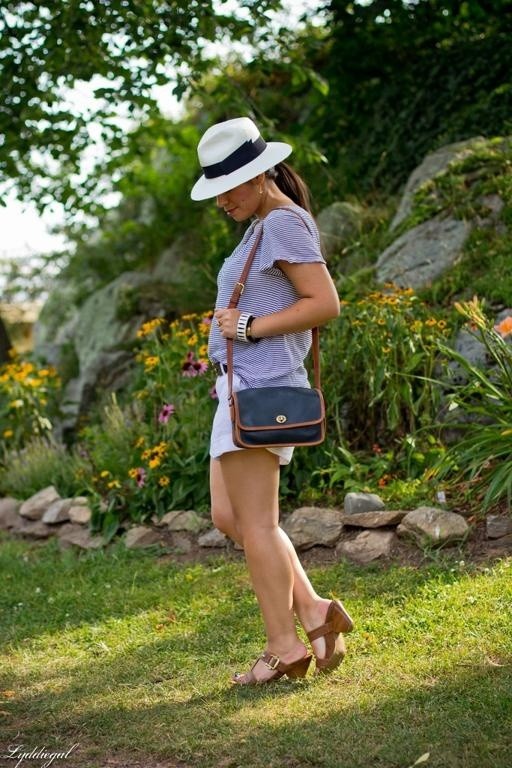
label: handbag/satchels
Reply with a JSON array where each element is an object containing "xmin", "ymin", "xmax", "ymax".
[{"xmin": 229, "ymin": 383, "xmax": 332, "ymax": 452}]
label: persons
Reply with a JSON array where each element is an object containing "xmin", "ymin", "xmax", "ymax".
[{"xmin": 185, "ymin": 114, "xmax": 353, "ymax": 690}]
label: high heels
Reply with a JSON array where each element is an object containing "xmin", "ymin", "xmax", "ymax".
[
  {"xmin": 231, "ymin": 648, "xmax": 313, "ymax": 687},
  {"xmin": 306, "ymin": 595, "xmax": 356, "ymax": 680}
]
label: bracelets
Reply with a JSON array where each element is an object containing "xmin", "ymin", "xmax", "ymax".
[{"xmin": 236, "ymin": 308, "xmax": 262, "ymax": 346}]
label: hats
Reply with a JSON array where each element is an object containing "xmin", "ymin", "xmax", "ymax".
[{"xmin": 186, "ymin": 113, "xmax": 297, "ymax": 205}]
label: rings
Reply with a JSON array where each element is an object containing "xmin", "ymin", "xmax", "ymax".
[{"xmin": 218, "ymin": 320, "xmax": 222, "ymax": 326}]
